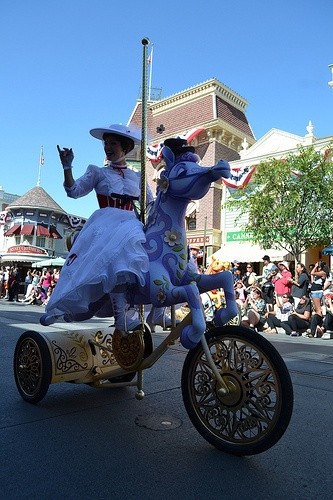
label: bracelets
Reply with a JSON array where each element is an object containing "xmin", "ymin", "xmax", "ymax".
[{"xmin": 63, "ymin": 166, "xmax": 72, "ymax": 170}]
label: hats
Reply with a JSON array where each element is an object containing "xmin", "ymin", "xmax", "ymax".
[
  {"xmin": 277, "ymin": 261, "xmax": 290, "ymax": 270},
  {"xmin": 89, "ymin": 123, "xmax": 141, "ymax": 145},
  {"xmin": 190, "ymin": 243, "xmax": 201, "ymax": 250},
  {"xmin": 324, "ymin": 294, "xmax": 333, "ymax": 298}
]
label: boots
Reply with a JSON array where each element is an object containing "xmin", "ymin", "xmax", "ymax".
[
  {"xmin": 146, "ymin": 306, "xmax": 181, "ymax": 333},
  {"xmin": 110, "ymin": 290, "xmax": 143, "ymax": 337}
]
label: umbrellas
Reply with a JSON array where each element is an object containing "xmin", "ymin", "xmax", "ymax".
[{"xmin": 32, "ymin": 256, "xmax": 66, "ymax": 268}]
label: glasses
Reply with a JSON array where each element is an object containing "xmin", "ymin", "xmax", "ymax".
[
  {"xmin": 301, "ymin": 297, "xmax": 305, "ymax": 300},
  {"xmin": 282, "ymin": 296, "xmax": 288, "ymax": 298}
]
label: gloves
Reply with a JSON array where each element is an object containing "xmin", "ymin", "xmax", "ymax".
[{"xmin": 56, "ymin": 144, "xmax": 75, "ymax": 170}]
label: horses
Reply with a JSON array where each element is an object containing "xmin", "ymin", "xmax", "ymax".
[{"xmin": 38, "ymin": 146, "xmax": 240, "ymax": 350}]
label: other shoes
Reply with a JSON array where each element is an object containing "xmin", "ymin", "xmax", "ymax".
[
  {"xmin": 263, "ymin": 327, "xmax": 272, "ymax": 333},
  {"xmin": 267, "ymin": 328, "xmax": 278, "ymax": 334},
  {"xmin": 317, "ymin": 325, "xmax": 324, "ymax": 335},
  {"xmin": 291, "ymin": 331, "xmax": 301, "ymax": 336},
  {"xmin": 306, "ymin": 333, "xmax": 314, "ymax": 338}
]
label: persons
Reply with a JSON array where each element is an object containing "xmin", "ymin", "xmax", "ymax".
[
  {"xmin": 45, "ymin": 123, "xmax": 181, "ymax": 331},
  {"xmin": 188, "ymin": 242, "xmax": 333, "ymax": 341},
  {"xmin": 0, "ymin": 263, "xmax": 60, "ymax": 307}
]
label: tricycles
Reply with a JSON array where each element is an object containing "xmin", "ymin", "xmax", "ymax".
[
  {"xmin": 12, "ymin": 37, "xmax": 293, "ymax": 457},
  {"xmin": 169, "ymin": 217, "xmax": 243, "ymax": 326}
]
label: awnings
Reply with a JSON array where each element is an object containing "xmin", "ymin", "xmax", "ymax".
[
  {"xmin": 20, "ymin": 224, "xmax": 35, "ymax": 236},
  {"xmin": 50, "ymin": 225, "xmax": 63, "ymax": 239},
  {"xmin": 37, "ymin": 223, "xmax": 49, "ymax": 237},
  {"xmin": 4, "ymin": 225, "xmax": 21, "ymax": 236},
  {"xmin": 185, "ymin": 201, "xmax": 196, "ymax": 218},
  {"xmin": 208, "ymin": 243, "xmax": 297, "ymax": 261}
]
